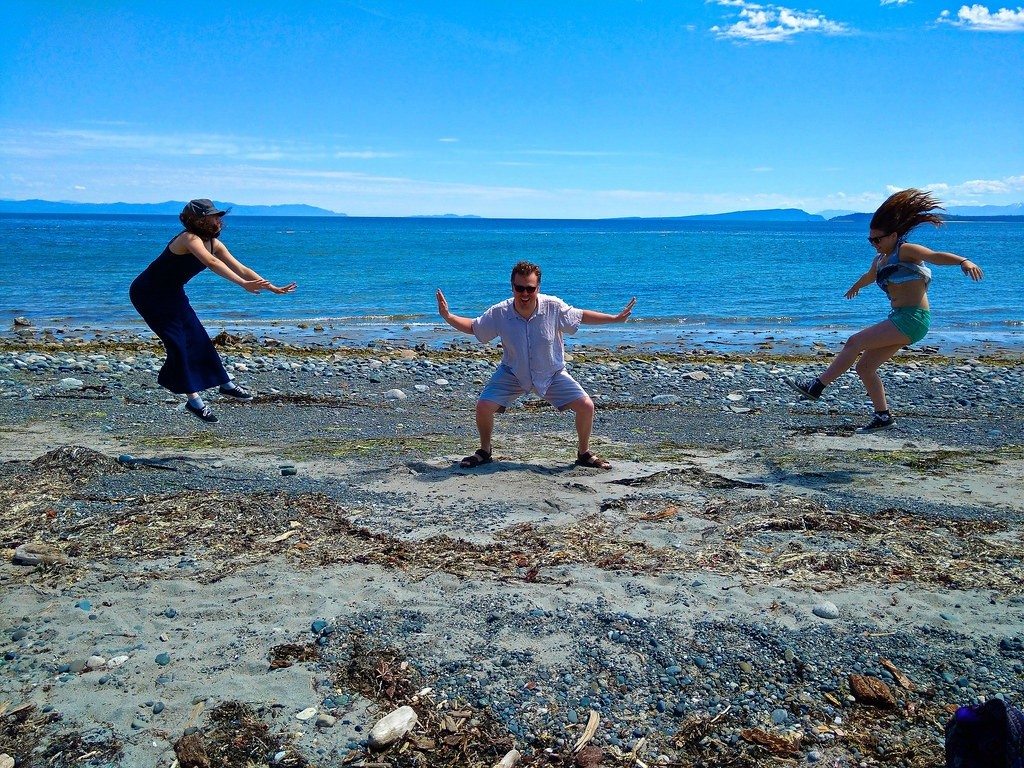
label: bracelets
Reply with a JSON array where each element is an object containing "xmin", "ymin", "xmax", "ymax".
[{"xmin": 959, "ymin": 258, "xmax": 969, "ymax": 265}]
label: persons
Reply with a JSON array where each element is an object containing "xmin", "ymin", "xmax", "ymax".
[
  {"xmin": 129, "ymin": 198, "xmax": 298, "ymax": 425},
  {"xmin": 785, "ymin": 188, "xmax": 984, "ymax": 435},
  {"xmin": 436, "ymin": 261, "xmax": 636, "ymax": 470}
]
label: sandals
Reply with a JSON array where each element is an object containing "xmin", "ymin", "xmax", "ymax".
[
  {"xmin": 459, "ymin": 448, "xmax": 492, "ymax": 469},
  {"xmin": 575, "ymin": 450, "xmax": 612, "ymax": 469}
]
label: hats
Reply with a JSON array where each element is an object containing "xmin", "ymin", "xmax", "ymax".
[{"xmin": 179, "ymin": 199, "xmax": 226, "ymax": 217}]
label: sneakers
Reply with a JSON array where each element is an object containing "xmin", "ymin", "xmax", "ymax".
[
  {"xmin": 783, "ymin": 376, "xmax": 826, "ymax": 402},
  {"xmin": 219, "ymin": 384, "xmax": 254, "ymax": 402},
  {"xmin": 185, "ymin": 401, "xmax": 220, "ymax": 424},
  {"xmin": 854, "ymin": 408, "xmax": 898, "ymax": 434}
]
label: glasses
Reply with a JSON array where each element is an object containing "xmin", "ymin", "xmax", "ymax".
[
  {"xmin": 513, "ymin": 282, "xmax": 539, "ymax": 294},
  {"xmin": 867, "ymin": 234, "xmax": 891, "ymax": 244}
]
